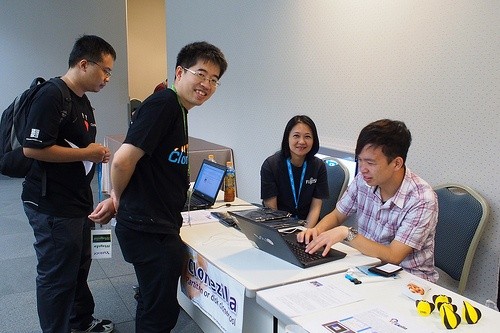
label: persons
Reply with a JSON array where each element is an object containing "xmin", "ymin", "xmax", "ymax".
[
  {"xmin": 261, "ymin": 115, "xmax": 329, "ymax": 228},
  {"xmin": 88, "ymin": 41, "xmax": 228, "ymax": 333},
  {"xmin": 21, "ymin": 34, "xmax": 117, "ymax": 333},
  {"xmin": 296, "ymin": 119, "xmax": 440, "ymax": 286}
]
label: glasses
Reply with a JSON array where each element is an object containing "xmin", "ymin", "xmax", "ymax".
[
  {"xmin": 182, "ymin": 64, "xmax": 221, "ymax": 88},
  {"xmin": 88, "ymin": 59, "xmax": 113, "ymax": 79}
]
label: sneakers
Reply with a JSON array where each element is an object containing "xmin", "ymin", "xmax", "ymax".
[{"xmin": 73, "ymin": 318, "xmax": 115, "ymax": 333}]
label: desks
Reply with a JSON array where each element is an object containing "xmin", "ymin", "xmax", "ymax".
[
  {"xmin": 256, "ymin": 271, "xmax": 500, "ymax": 333},
  {"xmin": 176, "ymin": 182, "xmax": 382, "ymax": 333},
  {"xmin": 100, "ymin": 134, "xmax": 237, "ymax": 195}
]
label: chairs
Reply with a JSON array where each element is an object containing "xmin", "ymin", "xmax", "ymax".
[
  {"xmin": 321, "ymin": 157, "xmax": 349, "ymax": 218},
  {"xmin": 432, "ymin": 181, "xmax": 489, "ymax": 295}
]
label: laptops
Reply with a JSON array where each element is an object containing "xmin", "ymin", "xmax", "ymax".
[
  {"xmin": 181, "ymin": 159, "xmax": 227, "ymax": 213},
  {"xmin": 227, "ymin": 211, "xmax": 348, "ymax": 268}
]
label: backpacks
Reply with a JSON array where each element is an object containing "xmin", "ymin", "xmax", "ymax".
[{"xmin": 0, "ymin": 72, "xmax": 72, "ymax": 178}]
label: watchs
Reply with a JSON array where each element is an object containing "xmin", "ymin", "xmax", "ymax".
[{"xmin": 343, "ymin": 226, "xmax": 358, "ymax": 242}]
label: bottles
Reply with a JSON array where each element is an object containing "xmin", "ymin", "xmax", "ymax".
[
  {"xmin": 223, "ymin": 162, "xmax": 235, "ymax": 202},
  {"xmin": 208, "ymin": 154, "xmax": 217, "ymax": 163}
]
